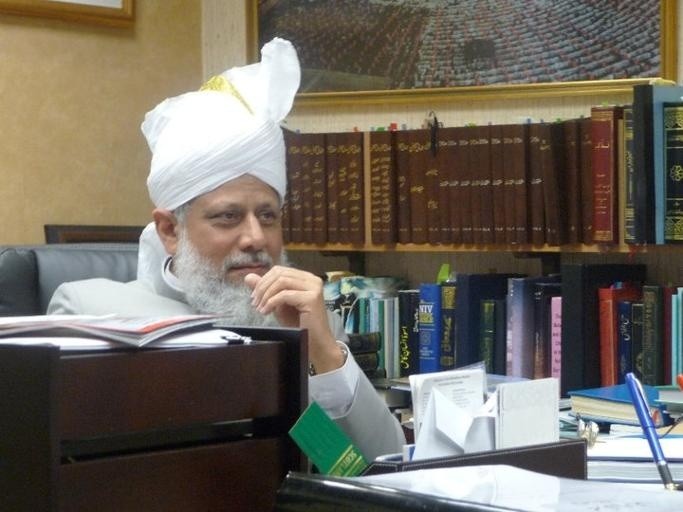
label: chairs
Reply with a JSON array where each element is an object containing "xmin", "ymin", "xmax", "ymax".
[{"xmin": 1, "ymin": 240, "xmax": 139, "ymax": 320}]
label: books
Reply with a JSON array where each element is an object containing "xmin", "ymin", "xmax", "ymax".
[
  {"xmin": 0, "ymin": 311, "xmax": 219, "ymax": 350},
  {"xmin": 276, "ymin": 83, "xmax": 682, "ymax": 482}
]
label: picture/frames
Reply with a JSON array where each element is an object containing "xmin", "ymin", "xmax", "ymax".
[
  {"xmin": 0, "ymin": 0, "xmax": 138, "ymax": 29},
  {"xmin": 244, "ymin": 0, "xmax": 677, "ymax": 108}
]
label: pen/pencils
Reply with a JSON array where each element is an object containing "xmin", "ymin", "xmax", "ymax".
[{"xmin": 624, "ymin": 372, "xmax": 677, "ymax": 491}]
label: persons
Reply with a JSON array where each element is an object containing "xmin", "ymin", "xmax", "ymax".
[{"xmin": 34, "ymin": 35, "xmax": 407, "ymax": 465}]
